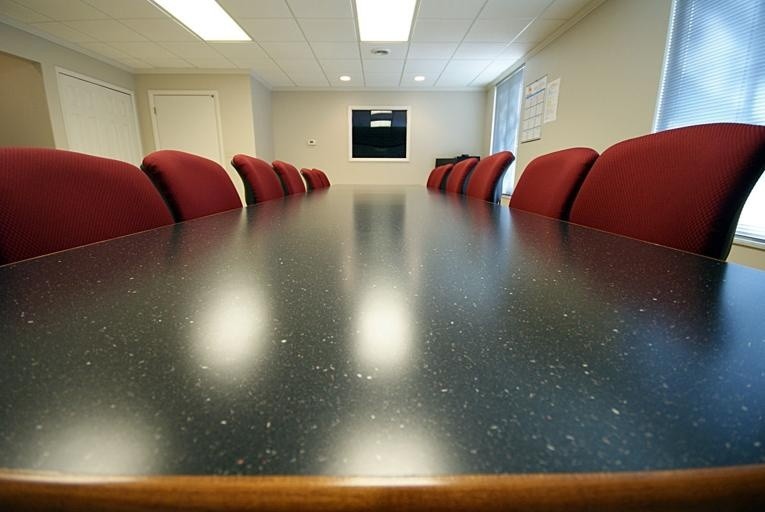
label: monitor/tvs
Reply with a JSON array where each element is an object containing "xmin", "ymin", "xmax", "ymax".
[{"xmin": 348, "ymin": 105, "xmax": 410, "ymax": 162}]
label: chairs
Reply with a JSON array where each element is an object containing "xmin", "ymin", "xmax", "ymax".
[
  {"xmin": 463, "ymin": 150, "xmax": 515, "ymax": 203},
  {"xmin": 1, "ymin": 146, "xmax": 174, "ymax": 266},
  {"xmin": 272, "ymin": 160, "xmax": 305, "ymax": 196},
  {"xmin": 509, "ymin": 148, "xmax": 600, "ymax": 222},
  {"xmin": 569, "ymin": 122, "xmax": 765, "ymax": 260},
  {"xmin": 140, "ymin": 150, "xmax": 243, "ymax": 223},
  {"xmin": 426, "ymin": 163, "xmax": 454, "ymax": 190},
  {"xmin": 446, "ymin": 158, "xmax": 479, "ymax": 194},
  {"xmin": 230, "ymin": 153, "xmax": 285, "ymax": 206},
  {"xmin": 300, "ymin": 168, "xmax": 330, "ymax": 191}
]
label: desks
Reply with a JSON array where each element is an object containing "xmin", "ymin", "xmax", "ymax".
[{"xmin": 1, "ymin": 184, "xmax": 763, "ymax": 511}]
